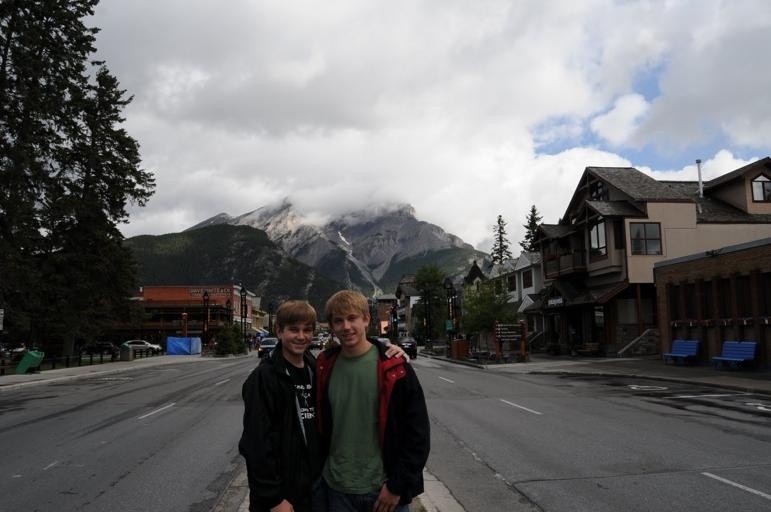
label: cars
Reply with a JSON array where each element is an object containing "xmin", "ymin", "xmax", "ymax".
[
  {"xmin": 398, "ymin": 341, "xmax": 418, "ymax": 359},
  {"xmin": 311, "ymin": 332, "xmax": 329, "ymax": 350},
  {"xmin": 258, "ymin": 338, "xmax": 279, "ymax": 358},
  {"xmin": 85, "ymin": 338, "xmax": 163, "ymax": 360}
]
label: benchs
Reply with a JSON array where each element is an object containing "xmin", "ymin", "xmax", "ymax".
[{"xmin": 662, "ymin": 340, "xmax": 757, "ymax": 371}]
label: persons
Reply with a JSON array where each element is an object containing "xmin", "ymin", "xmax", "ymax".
[
  {"xmin": 238, "ymin": 301, "xmax": 414, "ymax": 512},
  {"xmin": 310, "ymin": 290, "xmax": 430, "ymax": 511}
]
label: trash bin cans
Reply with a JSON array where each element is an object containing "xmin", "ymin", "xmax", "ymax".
[{"xmin": 14, "ymin": 349, "xmax": 45, "ymax": 374}]
